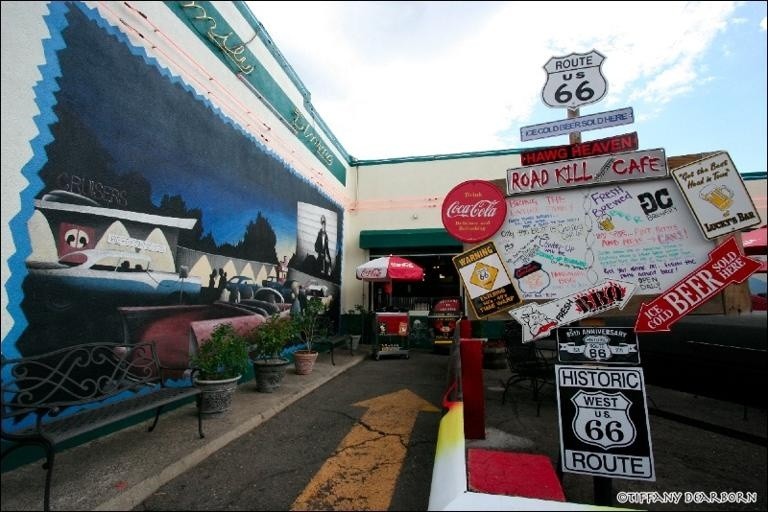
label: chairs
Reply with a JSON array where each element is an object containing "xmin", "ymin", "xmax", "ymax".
[{"xmin": 501, "ymin": 328, "xmax": 556, "ymax": 416}]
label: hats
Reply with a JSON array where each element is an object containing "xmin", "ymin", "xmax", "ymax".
[{"xmin": 321, "ymin": 215, "xmax": 326, "ymax": 224}]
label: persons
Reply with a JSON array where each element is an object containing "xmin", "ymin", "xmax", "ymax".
[{"xmin": 316, "ymin": 215, "xmax": 331, "ymax": 276}]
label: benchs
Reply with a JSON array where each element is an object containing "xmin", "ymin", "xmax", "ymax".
[
  {"xmin": 311, "ymin": 314, "xmax": 354, "ymax": 365},
  {"xmin": 0, "ymin": 338, "xmax": 205, "ymax": 512}
]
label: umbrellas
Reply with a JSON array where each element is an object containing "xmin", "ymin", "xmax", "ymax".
[{"xmin": 356, "ymin": 254, "xmax": 422, "ymax": 306}]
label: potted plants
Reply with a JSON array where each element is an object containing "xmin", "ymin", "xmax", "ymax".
[
  {"xmin": 187, "ymin": 297, "xmax": 330, "ymax": 421},
  {"xmin": 344, "ymin": 304, "xmax": 365, "ymax": 350}
]
